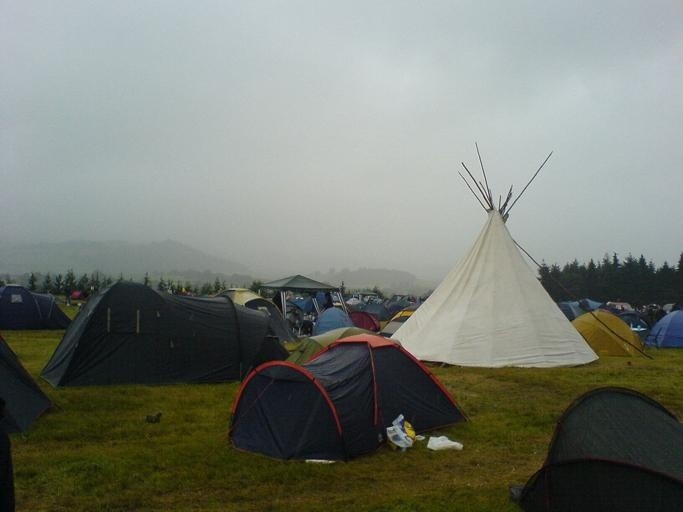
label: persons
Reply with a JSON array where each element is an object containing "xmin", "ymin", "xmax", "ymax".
[{"xmin": 64, "ymin": 282, "xmax": 99, "ymax": 306}]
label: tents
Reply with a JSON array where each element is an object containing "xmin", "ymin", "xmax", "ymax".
[
  {"xmin": 0, "ymin": 335, "xmax": 54, "ymax": 433},
  {"xmin": 390, "ymin": 210, "xmax": 599, "ymax": 369},
  {"xmin": 0, "ymin": 285, "xmax": 72, "ymax": 329},
  {"xmin": 40, "ymin": 281, "xmax": 291, "ymax": 388},
  {"xmin": 229, "ymin": 334, "xmax": 468, "ymax": 459},
  {"xmin": 215, "ymin": 275, "xmax": 429, "ymax": 351},
  {"xmin": 518, "ymin": 386, "xmax": 683, "ymax": 512},
  {"xmin": 559, "ymin": 299, "xmax": 683, "ymax": 357}
]
